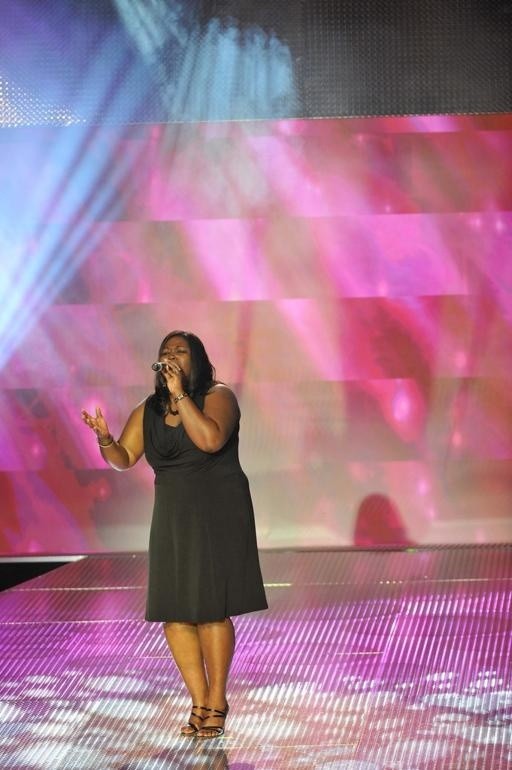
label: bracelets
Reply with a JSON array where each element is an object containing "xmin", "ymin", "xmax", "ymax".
[
  {"xmin": 173, "ymin": 390, "xmax": 191, "ymax": 403},
  {"xmin": 97, "ymin": 434, "xmax": 114, "ymax": 448}
]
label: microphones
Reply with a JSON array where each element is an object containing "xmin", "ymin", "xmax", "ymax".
[{"xmin": 152, "ymin": 362, "xmax": 167, "ymax": 372}]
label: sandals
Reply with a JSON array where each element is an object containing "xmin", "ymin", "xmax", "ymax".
[
  {"xmin": 178, "ymin": 706, "xmax": 207, "ymax": 736},
  {"xmin": 196, "ymin": 702, "xmax": 230, "ymax": 741}
]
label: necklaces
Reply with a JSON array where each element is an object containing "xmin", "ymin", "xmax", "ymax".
[{"xmin": 165, "ymin": 403, "xmax": 180, "ymax": 416}]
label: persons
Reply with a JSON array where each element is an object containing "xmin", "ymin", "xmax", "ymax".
[{"xmin": 81, "ymin": 331, "xmax": 268, "ymax": 738}]
